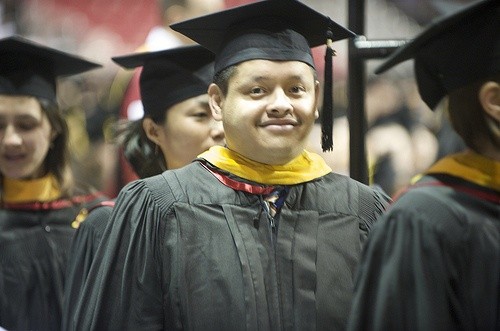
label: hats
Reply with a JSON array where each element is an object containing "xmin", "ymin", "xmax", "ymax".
[
  {"xmin": 0, "ymin": 38, "xmax": 102, "ymax": 99},
  {"xmin": 111, "ymin": 46, "xmax": 216, "ymax": 114},
  {"xmin": 375, "ymin": 0, "xmax": 500, "ymax": 110},
  {"xmin": 169, "ymin": 0, "xmax": 356, "ymax": 152}
]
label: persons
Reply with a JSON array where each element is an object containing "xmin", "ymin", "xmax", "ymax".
[
  {"xmin": 60, "ymin": 45, "xmax": 226, "ymax": 331},
  {"xmin": 73, "ymin": 0, "xmax": 390, "ymax": 331},
  {"xmin": 344, "ymin": 0, "xmax": 500, "ymax": 331},
  {"xmin": 0, "ymin": 36, "xmax": 110, "ymax": 331}
]
